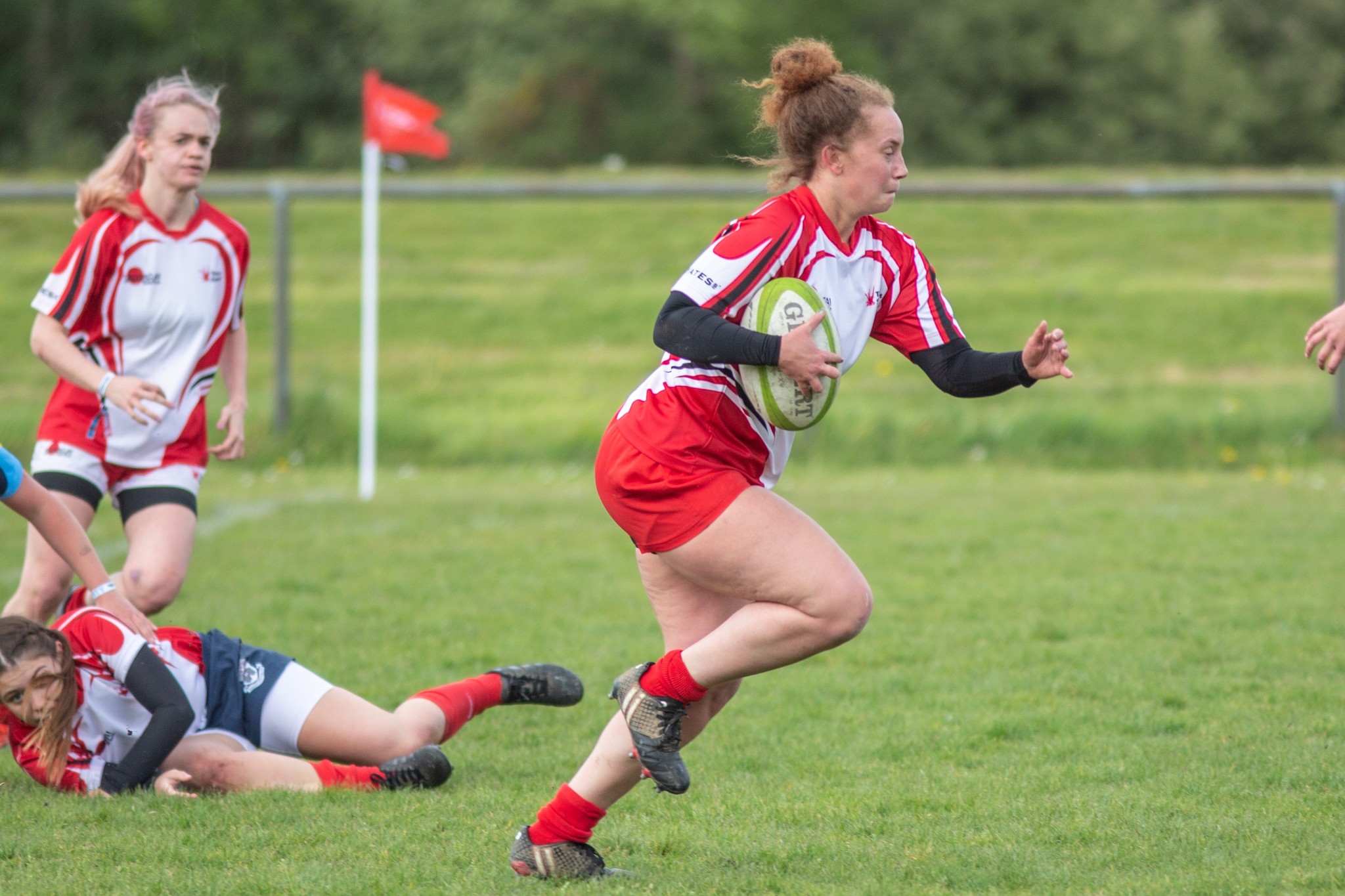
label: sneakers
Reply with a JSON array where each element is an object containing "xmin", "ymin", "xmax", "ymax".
[
  {"xmin": 370, "ymin": 745, "xmax": 452, "ymax": 790},
  {"xmin": 484, "ymin": 663, "xmax": 584, "ymax": 708},
  {"xmin": 509, "ymin": 825, "xmax": 636, "ymax": 885},
  {"xmin": 608, "ymin": 661, "xmax": 690, "ymax": 795}
]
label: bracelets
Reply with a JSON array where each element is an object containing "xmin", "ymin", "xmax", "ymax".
[
  {"xmin": 97, "ymin": 371, "xmax": 116, "ymax": 397},
  {"xmin": 91, "ymin": 581, "xmax": 116, "ymax": 599}
]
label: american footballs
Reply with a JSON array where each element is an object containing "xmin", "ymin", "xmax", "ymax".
[{"xmin": 738, "ymin": 278, "xmax": 842, "ymax": 432}]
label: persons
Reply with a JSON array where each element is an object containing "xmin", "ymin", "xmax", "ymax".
[
  {"xmin": 0, "ymin": 443, "xmax": 160, "ymax": 646},
  {"xmin": 0, "ymin": 67, "xmax": 251, "ymax": 628},
  {"xmin": 508, "ymin": 35, "xmax": 1073, "ymax": 881},
  {"xmin": 1304, "ymin": 302, "xmax": 1345, "ymax": 375},
  {"xmin": 0, "ymin": 605, "xmax": 585, "ymax": 801}
]
